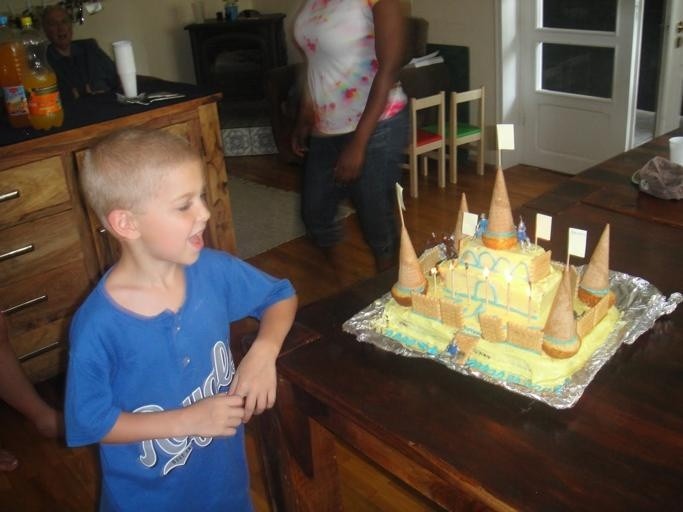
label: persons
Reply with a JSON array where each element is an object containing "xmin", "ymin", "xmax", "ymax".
[
  {"xmin": 290, "ymin": 0, "xmax": 411, "ymax": 289},
  {"xmin": 64, "ymin": 123, "xmax": 298, "ymax": 512},
  {"xmin": 0, "ymin": 312, "xmax": 66, "ymax": 472},
  {"xmin": 42, "ymin": 5, "xmax": 121, "ymax": 99}
]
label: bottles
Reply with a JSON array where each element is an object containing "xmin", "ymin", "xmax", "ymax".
[
  {"xmin": 0, "ymin": 16, "xmax": 33, "ymax": 130},
  {"xmin": 15, "ymin": 16, "xmax": 66, "ymax": 133}
]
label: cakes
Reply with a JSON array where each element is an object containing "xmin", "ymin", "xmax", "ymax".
[{"xmin": 381, "ymin": 122, "xmax": 620, "ymax": 386}]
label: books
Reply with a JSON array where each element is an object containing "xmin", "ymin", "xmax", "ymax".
[{"xmin": 404, "ymin": 50, "xmax": 444, "ymax": 68}]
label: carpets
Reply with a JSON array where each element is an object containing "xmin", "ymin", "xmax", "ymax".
[{"xmin": 226, "ymin": 171, "xmax": 356, "ymax": 261}]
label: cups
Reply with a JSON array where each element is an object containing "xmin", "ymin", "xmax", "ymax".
[
  {"xmin": 668, "ymin": 137, "xmax": 683, "ymax": 168},
  {"xmin": 111, "ymin": 40, "xmax": 137, "ymax": 99},
  {"xmin": 191, "ymin": 3, "xmax": 205, "ymax": 25}
]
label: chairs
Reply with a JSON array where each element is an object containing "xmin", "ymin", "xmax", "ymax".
[{"xmin": 399, "ymin": 85, "xmax": 485, "ymax": 198}]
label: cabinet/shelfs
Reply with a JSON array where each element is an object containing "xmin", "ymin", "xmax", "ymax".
[
  {"xmin": 184, "ymin": 13, "xmax": 289, "ymax": 125},
  {"xmin": 1, "ymin": 75, "xmax": 235, "ymax": 388}
]
